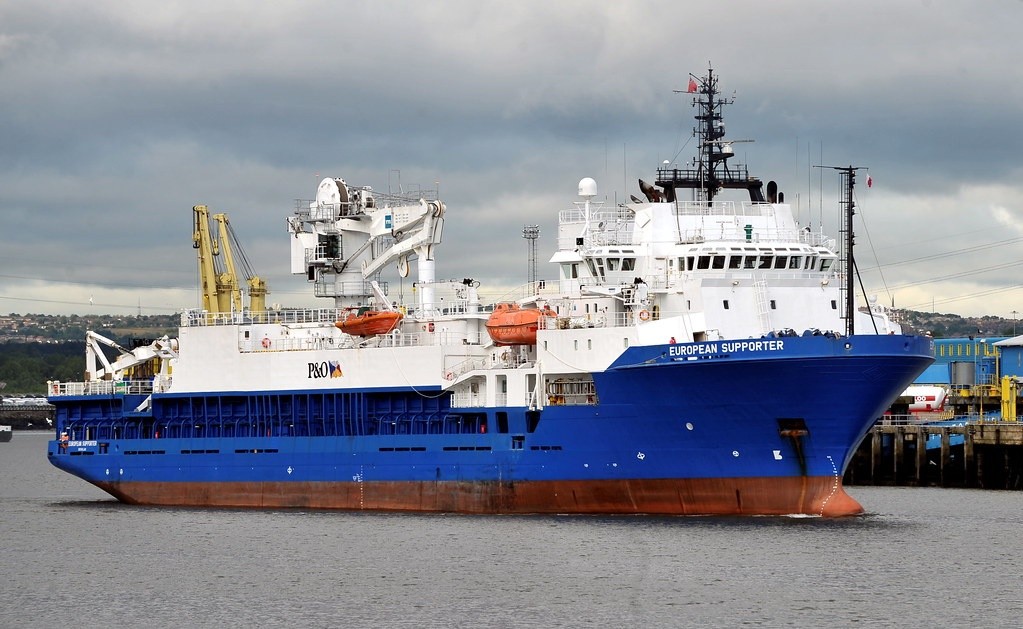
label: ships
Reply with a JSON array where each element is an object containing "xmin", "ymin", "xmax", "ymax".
[{"xmin": 47, "ymin": 63, "xmax": 938, "ymax": 523}]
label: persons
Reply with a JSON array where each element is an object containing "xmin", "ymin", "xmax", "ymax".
[
  {"xmin": 670, "ymin": 336, "xmax": 676, "ymax": 345},
  {"xmin": 866, "ymin": 175, "xmax": 872, "ymax": 188},
  {"xmin": 59, "ymin": 432, "xmax": 69, "ymax": 453},
  {"xmin": 588, "ymin": 395, "xmax": 594, "ymax": 403},
  {"xmin": 688, "ymin": 78, "xmax": 697, "ymax": 94}
]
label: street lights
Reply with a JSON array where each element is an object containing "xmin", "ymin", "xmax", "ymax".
[
  {"xmin": 1010, "ymin": 311, "xmax": 1019, "ymax": 338},
  {"xmin": 521, "ymin": 223, "xmax": 544, "ymax": 297}
]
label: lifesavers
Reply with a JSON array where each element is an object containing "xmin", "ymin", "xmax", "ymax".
[
  {"xmin": 262, "ymin": 338, "xmax": 272, "ymax": 348},
  {"xmin": 640, "ymin": 309, "xmax": 650, "ymax": 321}
]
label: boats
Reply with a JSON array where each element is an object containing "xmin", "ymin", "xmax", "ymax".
[
  {"xmin": 335, "ymin": 305, "xmax": 404, "ymax": 339},
  {"xmin": 483, "ymin": 301, "xmax": 559, "ymax": 346}
]
